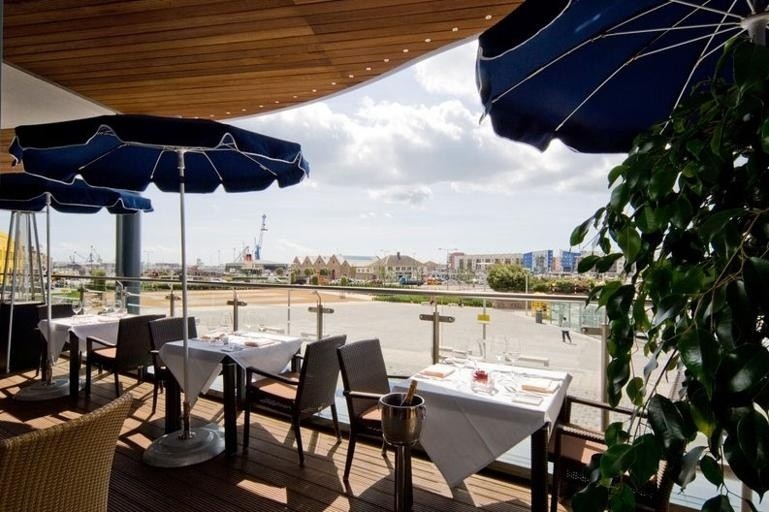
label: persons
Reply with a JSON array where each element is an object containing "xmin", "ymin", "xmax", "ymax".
[{"xmin": 560, "ymin": 316, "xmax": 572, "ymax": 343}]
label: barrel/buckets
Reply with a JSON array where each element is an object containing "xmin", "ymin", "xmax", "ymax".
[{"xmin": 375, "ymin": 392, "xmax": 426, "ymax": 448}]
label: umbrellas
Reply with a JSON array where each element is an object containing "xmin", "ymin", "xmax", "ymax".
[
  {"xmin": 1, "ymin": 170, "xmax": 155, "ymax": 383},
  {"xmin": 6, "ymin": 103, "xmax": 313, "ymax": 440},
  {"xmin": 473, "ymin": 1, "xmax": 768, "ymax": 161}
]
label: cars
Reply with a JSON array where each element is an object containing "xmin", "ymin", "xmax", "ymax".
[
  {"xmin": 271, "ymin": 276, "xmax": 383, "ymax": 285},
  {"xmin": 27, "ymin": 277, "xmax": 67, "ymax": 294},
  {"xmin": 210, "ymin": 277, "xmax": 227, "ymax": 283},
  {"xmin": 395, "ymin": 277, "xmax": 486, "ymax": 286}
]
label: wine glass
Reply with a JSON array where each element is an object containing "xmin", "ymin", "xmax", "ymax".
[
  {"xmin": 72, "ymin": 298, "xmax": 92, "ymax": 324},
  {"xmin": 244, "ymin": 312, "xmax": 267, "ymax": 342},
  {"xmin": 505, "ymin": 336, "xmax": 522, "ymax": 382},
  {"xmin": 102, "ymin": 297, "xmax": 122, "ymax": 322}
]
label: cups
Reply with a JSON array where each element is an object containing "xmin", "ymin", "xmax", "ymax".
[
  {"xmin": 220, "ymin": 311, "xmax": 234, "ymax": 328},
  {"xmin": 452, "ymin": 344, "xmax": 468, "ymax": 365}
]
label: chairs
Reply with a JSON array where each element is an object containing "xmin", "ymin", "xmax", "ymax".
[
  {"xmin": 87, "ymin": 312, "xmax": 166, "ymax": 401},
  {"xmin": 241, "ymin": 333, "xmax": 346, "ymax": 467},
  {"xmin": 335, "ymin": 338, "xmax": 396, "ymax": 483},
  {"xmin": 34, "ymin": 303, "xmax": 82, "ymax": 376},
  {"xmin": 3, "ymin": 390, "xmax": 133, "ymax": 512},
  {"xmin": 149, "ymin": 316, "xmax": 225, "ymax": 412},
  {"xmin": 548, "ymin": 363, "xmax": 690, "ymax": 512}
]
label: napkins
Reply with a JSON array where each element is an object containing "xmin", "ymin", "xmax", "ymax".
[
  {"xmin": 520, "ymin": 378, "xmax": 558, "ymax": 392},
  {"xmin": 421, "ymin": 363, "xmax": 454, "ymax": 376},
  {"xmin": 244, "ymin": 339, "xmax": 274, "ymax": 346},
  {"xmin": 200, "ymin": 334, "xmax": 216, "ymax": 339}
]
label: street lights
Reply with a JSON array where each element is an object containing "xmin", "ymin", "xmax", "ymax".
[
  {"xmin": 517, "ymin": 271, "xmax": 534, "ymax": 315},
  {"xmin": 437, "ymin": 247, "xmax": 458, "ymax": 291}
]
